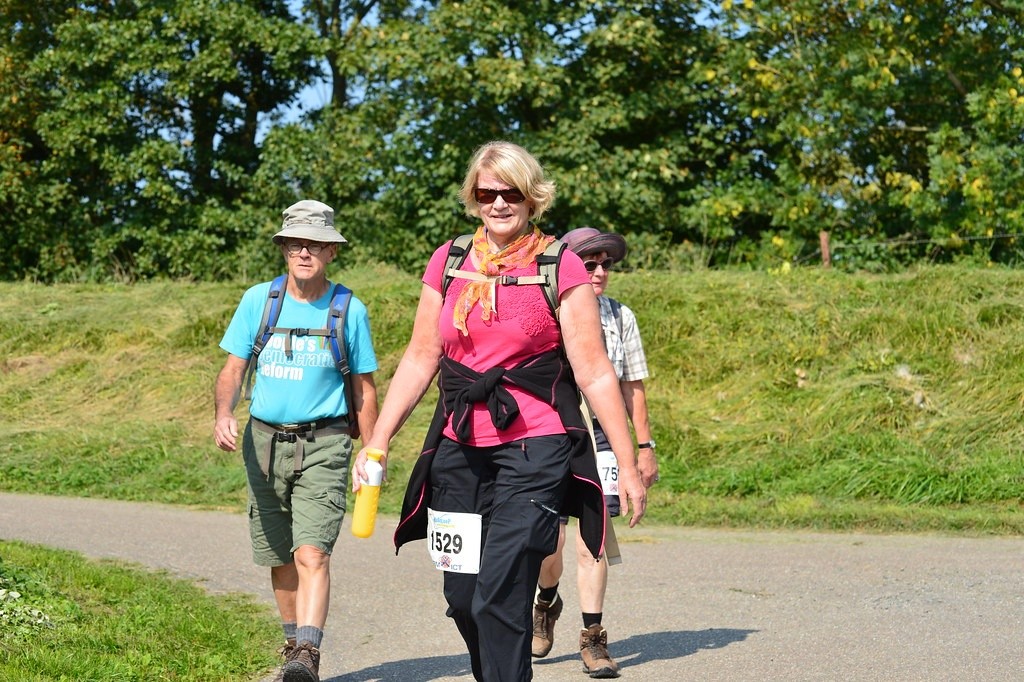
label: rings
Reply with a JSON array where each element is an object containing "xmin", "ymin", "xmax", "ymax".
[{"xmin": 655, "ymin": 477, "xmax": 659, "ymax": 481}]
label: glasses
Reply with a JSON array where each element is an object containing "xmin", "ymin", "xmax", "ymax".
[
  {"xmin": 582, "ymin": 257, "xmax": 615, "ymax": 272},
  {"xmin": 283, "ymin": 240, "xmax": 332, "ymax": 256},
  {"xmin": 474, "ymin": 188, "xmax": 525, "ymax": 204}
]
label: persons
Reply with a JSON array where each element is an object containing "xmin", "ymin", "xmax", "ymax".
[
  {"xmin": 213, "ymin": 200, "xmax": 379, "ymax": 682},
  {"xmin": 352, "ymin": 142, "xmax": 648, "ymax": 681},
  {"xmin": 532, "ymin": 227, "xmax": 660, "ymax": 678}
]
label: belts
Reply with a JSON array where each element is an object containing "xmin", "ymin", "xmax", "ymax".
[{"xmin": 260, "ymin": 417, "xmax": 345, "ymax": 436}]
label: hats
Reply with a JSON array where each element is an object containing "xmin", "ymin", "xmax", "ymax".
[
  {"xmin": 560, "ymin": 228, "xmax": 627, "ymax": 263},
  {"xmin": 271, "ymin": 200, "xmax": 348, "ymax": 242}
]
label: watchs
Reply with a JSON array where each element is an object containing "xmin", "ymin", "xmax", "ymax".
[{"xmin": 638, "ymin": 439, "xmax": 656, "ymax": 449}]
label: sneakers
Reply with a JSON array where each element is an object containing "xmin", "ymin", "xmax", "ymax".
[
  {"xmin": 282, "ymin": 642, "xmax": 320, "ymax": 682},
  {"xmin": 272, "ymin": 640, "xmax": 297, "ymax": 682},
  {"xmin": 579, "ymin": 623, "xmax": 617, "ymax": 678},
  {"xmin": 532, "ymin": 591, "xmax": 564, "ymax": 658}
]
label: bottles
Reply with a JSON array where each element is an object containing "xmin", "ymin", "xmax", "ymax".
[{"xmin": 352, "ymin": 449, "xmax": 384, "ymax": 538}]
